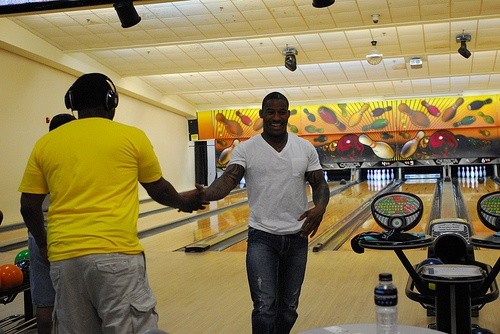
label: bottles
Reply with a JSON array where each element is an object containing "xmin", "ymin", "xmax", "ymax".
[{"xmin": 374, "ymin": 273, "xmax": 398, "ymax": 334}]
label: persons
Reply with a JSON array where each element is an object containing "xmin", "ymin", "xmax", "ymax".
[
  {"xmin": 16, "ymin": 72, "xmax": 209, "ymax": 334},
  {"xmin": 26, "ymin": 114, "xmax": 80, "ymax": 333},
  {"xmin": 195, "ymin": 90, "xmax": 330, "ymax": 334}
]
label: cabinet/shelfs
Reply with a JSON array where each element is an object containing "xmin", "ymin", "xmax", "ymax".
[{"xmin": 0, "ymin": 260, "xmax": 38, "ymax": 334}]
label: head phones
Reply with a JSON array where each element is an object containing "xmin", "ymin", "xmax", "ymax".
[{"xmin": 65, "ymin": 73, "xmax": 119, "ymax": 111}]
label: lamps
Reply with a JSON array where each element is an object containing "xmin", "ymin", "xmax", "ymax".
[
  {"xmin": 282, "ymin": 45, "xmax": 299, "ymax": 72},
  {"xmin": 456, "ymin": 34, "xmax": 472, "ymax": 59},
  {"xmin": 113, "ymin": 0, "xmax": 142, "ymax": 29},
  {"xmin": 366, "ymin": 54, "xmax": 383, "ymax": 66}
]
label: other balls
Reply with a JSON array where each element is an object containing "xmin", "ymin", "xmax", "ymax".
[
  {"xmin": 0, "ymin": 264, "xmax": 23, "ymax": 293},
  {"xmin": 14, "ymin": 250, "xmax": 29, "ymax": 270}
]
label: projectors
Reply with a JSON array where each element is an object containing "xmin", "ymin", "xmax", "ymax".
[{"xmin": 410, "ymin": 58, "xmax": 423, "ymax": 68}]
